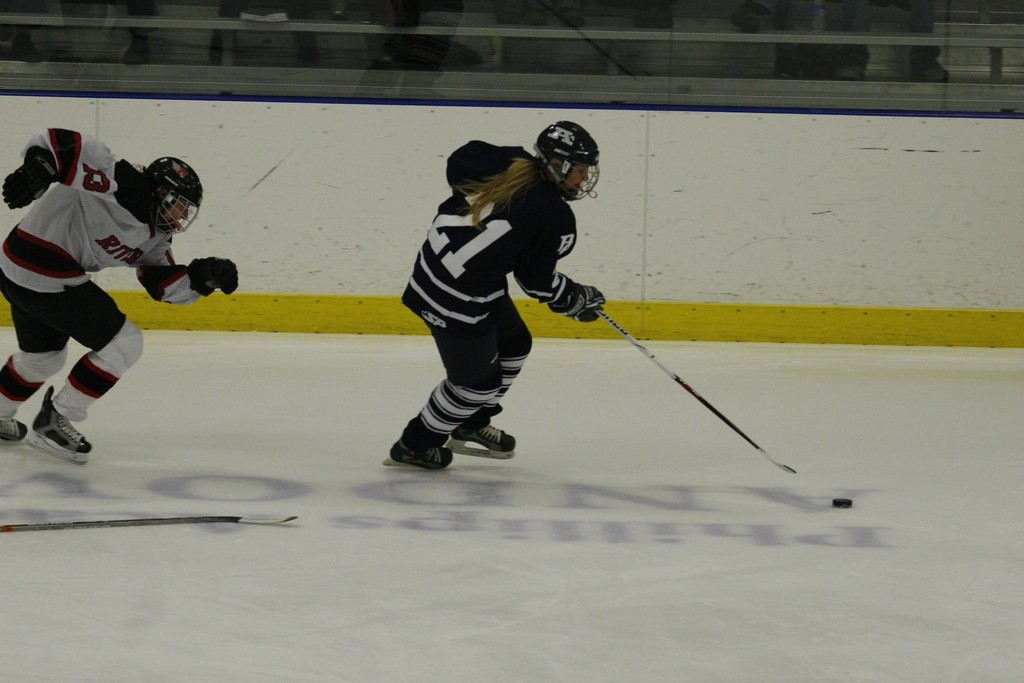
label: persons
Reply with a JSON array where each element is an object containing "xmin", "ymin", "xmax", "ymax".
[
  {"xmin": 0, "ymin": 128, "xmax": 238, "ymax": 465},
  {"xmin": 382, "ymin": 121, "xmax": 610, "ymax": 470},
  {"xmin": 1, "ymin": 0, "xmax": 959, "ymax": 84}
]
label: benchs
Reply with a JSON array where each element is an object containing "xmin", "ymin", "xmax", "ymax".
[{"xmin": 0, "ymin": 0, "xmax": 1024, "ymax": 113}]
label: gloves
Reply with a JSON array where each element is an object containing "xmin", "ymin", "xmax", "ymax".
[
  {"xmin": 546, "ymin": 272, "xmax": 606, "ymax": 322},
  {"xmin": 2, "ymin": 146, "xmax": 58, "ymax": 209},
  {"xmin": 187, "ymin": 256, "xmax": 239, "ymax": 296}
]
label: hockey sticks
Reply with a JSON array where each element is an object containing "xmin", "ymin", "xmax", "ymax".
[
  {"xmin": 1, "ymin": 514, "xmax": 299, "ymax": 532},
  {"xmin": 594, "ymin": 309, "xmax": 797, "ymax": 476}
]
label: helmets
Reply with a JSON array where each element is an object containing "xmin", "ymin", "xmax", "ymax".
[
  {"xmin": 533, "ymin": 120, "xmax": 601, "ymax": 199},
  {"xmin": 141, "ymin": 156, "xmax": 203, "ymax": 235}
]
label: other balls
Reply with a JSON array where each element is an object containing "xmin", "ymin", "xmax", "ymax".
[{"xmin": 833, "ymin": 498, "xmax": 852, "ymax": 508}]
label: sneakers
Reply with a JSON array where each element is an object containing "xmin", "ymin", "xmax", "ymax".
[
  {"xmin": 447, "ymin": 404, "xmax": 516, "ymax": 459},
  {"xmin": 27, "ymin": 388, "xmax": 92, "ymax": 462},
  {"xmin": 0, "ymin": 416, "xmax": 28, "ymax": 442},
  {"xmin": 383, "ymin": 416, "xmax": 454, "ymax": 471}
]
